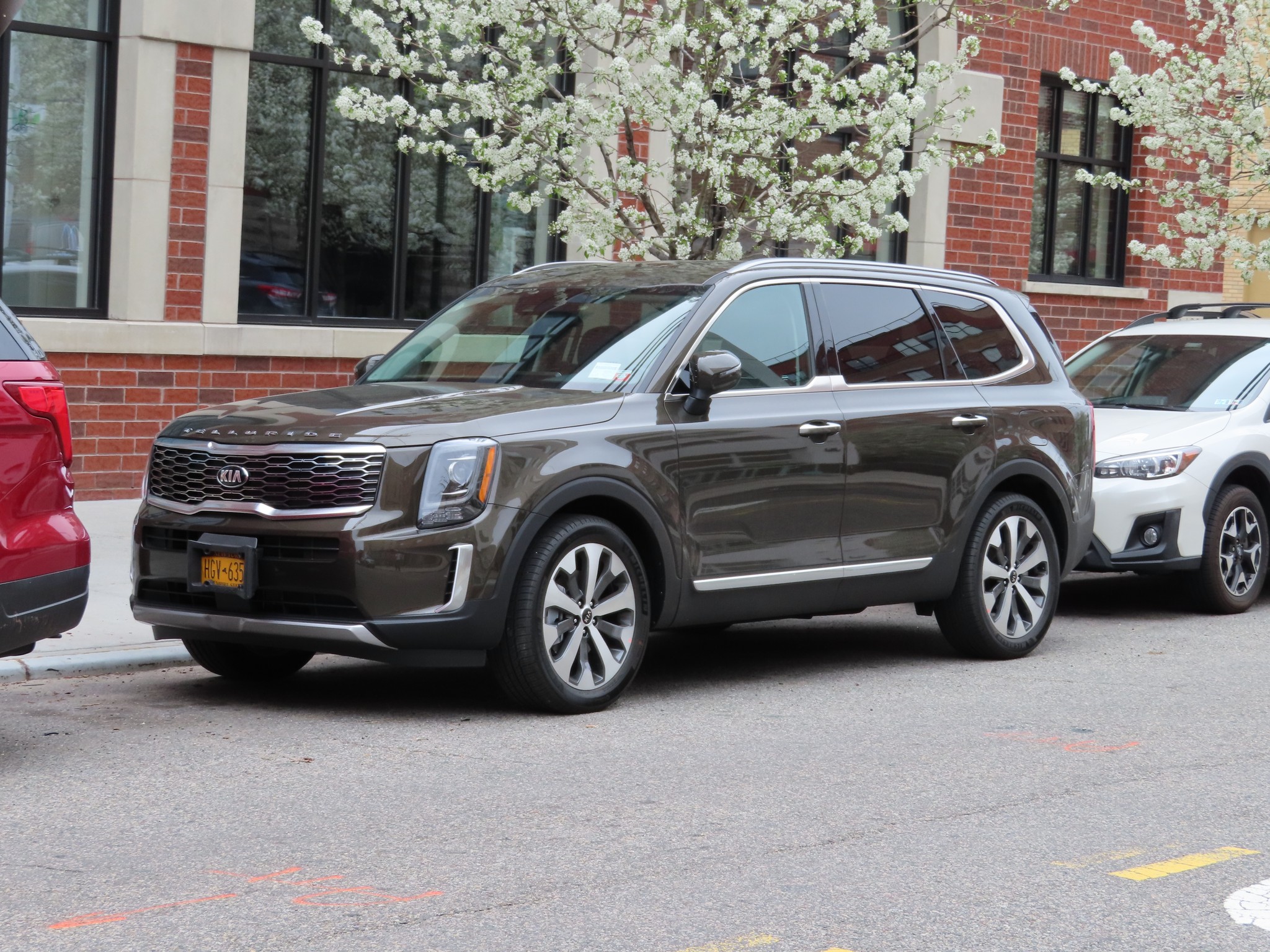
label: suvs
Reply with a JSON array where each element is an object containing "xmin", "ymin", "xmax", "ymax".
[{"xmin": 130, "ymin": 258, "xmax": 1097, "ymax": 717}]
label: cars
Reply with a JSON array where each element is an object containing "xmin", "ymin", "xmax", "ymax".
[
  {"xmin": 0, "ymin": 304, "xmax": 92, "ymax": 658},
  {"xmin": 238, "ymin": 248, "xmax": 346, "ymax": 316},
  {"xmin": 1063, "ymin": 302, "xmax": 1270, "ymax": 611},
  {"xmin": 2, "ymin": 223, "xmax": 79, "ymax": 308}
]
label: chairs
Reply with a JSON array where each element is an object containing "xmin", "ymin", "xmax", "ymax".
[{"xmin": 559, "ymin": 326, "xmax": 626, "ymax": 375}]
